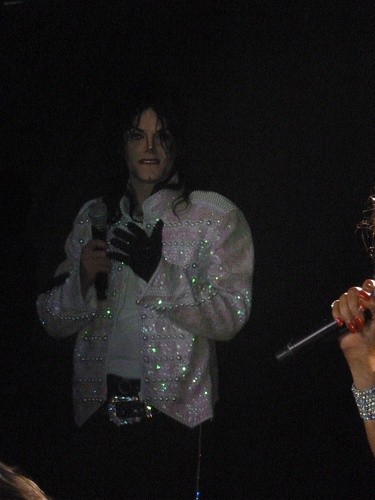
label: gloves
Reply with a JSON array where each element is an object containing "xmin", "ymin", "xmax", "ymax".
[{"xmin": 108, "ymin": 218, "xmax": 163, "ymax": 284}]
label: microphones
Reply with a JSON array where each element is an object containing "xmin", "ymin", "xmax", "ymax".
[
  {"xmin": 88, "ymin": 201, "xmax": 111, "ymax": 299},
  {"xmin": 275, "ymin": 306, "xmax": 373, "ymax": 364}
]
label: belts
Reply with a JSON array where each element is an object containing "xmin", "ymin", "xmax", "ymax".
[{"xmin": 96, "ymin": 395, "xmax": 160, "ymax": 425}]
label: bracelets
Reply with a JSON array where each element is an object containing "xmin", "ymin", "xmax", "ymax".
[{"xmin": 352, "ymin": 385, "xmax": 375, "ymax": 421}]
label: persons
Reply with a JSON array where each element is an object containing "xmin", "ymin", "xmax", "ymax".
[
  {"xmin": 0, "ymin": 462, "xmax": 52, "ymax": 500},
  {"xmin": 331, "ymin": 188, "xmax": 375, "ymax": 453},
  {"xmin": 36, "ymin": 80, "xmax": 255, "ymax": 500}
]
